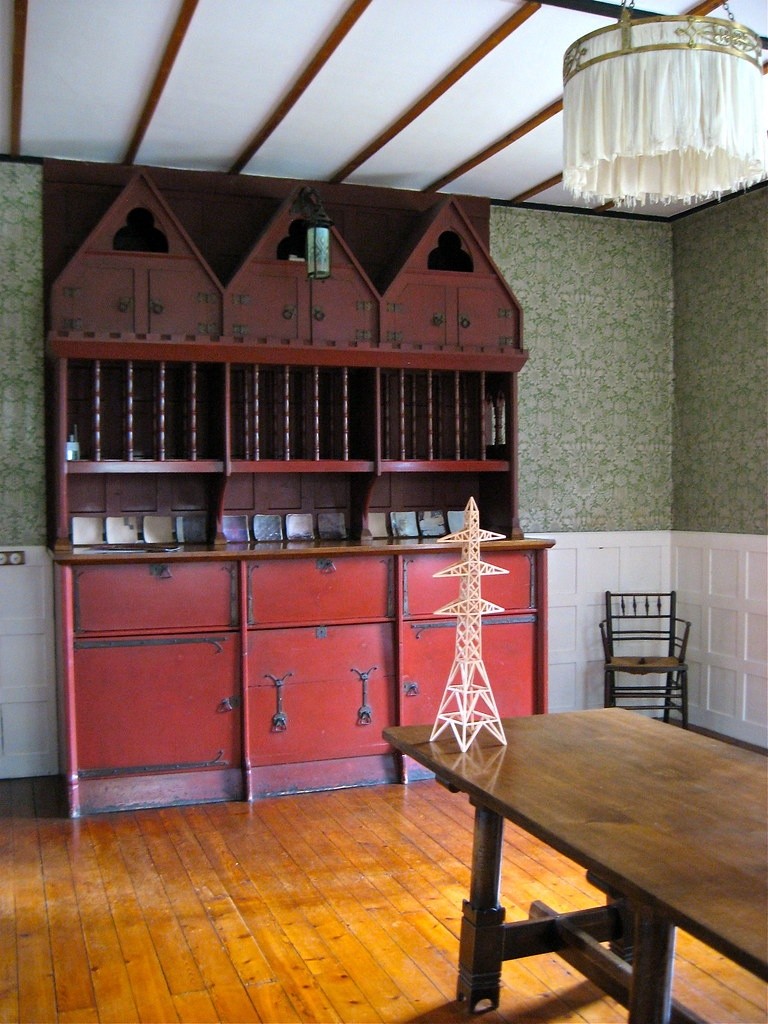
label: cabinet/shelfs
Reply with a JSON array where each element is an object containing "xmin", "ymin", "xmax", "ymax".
[
  {"xmin": 226, "ymin": 180, "xmax": 387, "ymax": 348},
  {"xmin": 227, "ymin": 346, "xmax": 380, "ymax": 545},
  {"xmin": 47, "ymin": 544, "xmax": 250, "ymax": 819},
  {"xmin": 50, "ymin": 163, "xmax": 227, "ymax": 345},
  {"xmin": 245, "ymin": 537, "xmax": 407, "ymax": 800},
  {"xmin": 401, "ymin": 534, "xmax": 556, "ymax": 784},
  {"xmin": 383, "ymin": 193, "xmax": 531, "ymax": 353},
  {"xmin": 378, "ymin": 350, "xmax": 529, "ymax": 541},
  {"xmin": 48, "ymin": 340, "xmax": 228, "ymax": 547}
]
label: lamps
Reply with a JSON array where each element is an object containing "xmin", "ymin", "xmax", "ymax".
[
  {"xmin": 558, "ymin": 0, "xmax": 768, "ymax": 209},
  {"xmin": 290, "ymin": 185, "xmax": 336, "ymax": 279}
]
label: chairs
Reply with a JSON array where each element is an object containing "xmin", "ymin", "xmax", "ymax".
[{"xmin": 598, "ymin": 590, "xmax": 691, "ymax": 731}]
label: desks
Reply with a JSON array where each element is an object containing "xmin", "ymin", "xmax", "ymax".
[{"xmin": 380, "ymin": 705, "xmax": 768, "ymax": 1024}]
